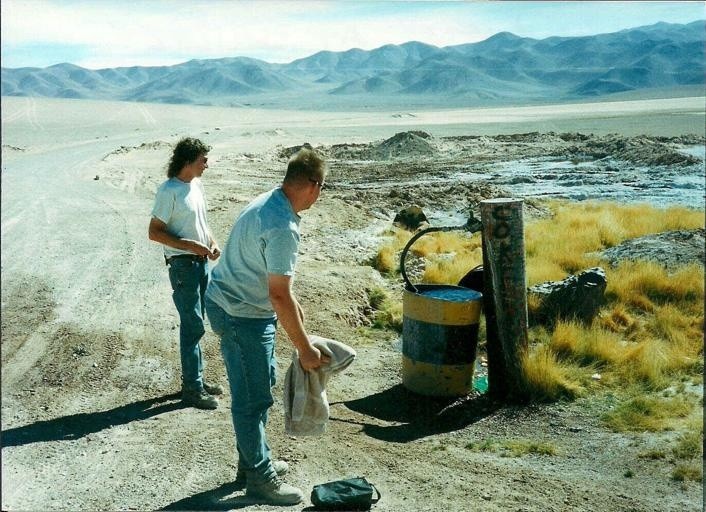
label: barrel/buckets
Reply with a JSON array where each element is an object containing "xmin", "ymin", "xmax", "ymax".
[{"xmin": 400, "ymin": 283, "xmax": 483, "ymax": 395}]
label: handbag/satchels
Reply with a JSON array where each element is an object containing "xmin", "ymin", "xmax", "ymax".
[{"xmin": 310, "ymin": 475, "xmax": 381, "ymax": 511}]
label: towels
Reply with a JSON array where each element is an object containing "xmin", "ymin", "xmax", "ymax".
[{"xmin": 283, "ymin": 335, "xmax": 356, "ymax": 437}]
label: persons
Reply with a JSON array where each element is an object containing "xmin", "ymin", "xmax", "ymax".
[
  {"xmin": 203, "ymin": 146, "xmax": 331, "ymax": 506},
  {"xmin": 149, "ymin": 136, "xmax": 221, "ymax": 410}
]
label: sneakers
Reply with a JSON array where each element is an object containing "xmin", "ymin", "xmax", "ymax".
[
  {"xmin": 244, "ymin": 477, "xmax": 304, "ymax": 504},
  {"xmin": 236, "ymin": 458, "xmax": 290, "ymax": 483},
  {"xmin": 180, "ymin": 383, "xmax": 223, "ymax": 409}
]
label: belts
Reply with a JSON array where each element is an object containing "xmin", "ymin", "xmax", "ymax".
[{"xmin": 172, "ymin": 254, "xmax": 207, "ymax": 259}]
